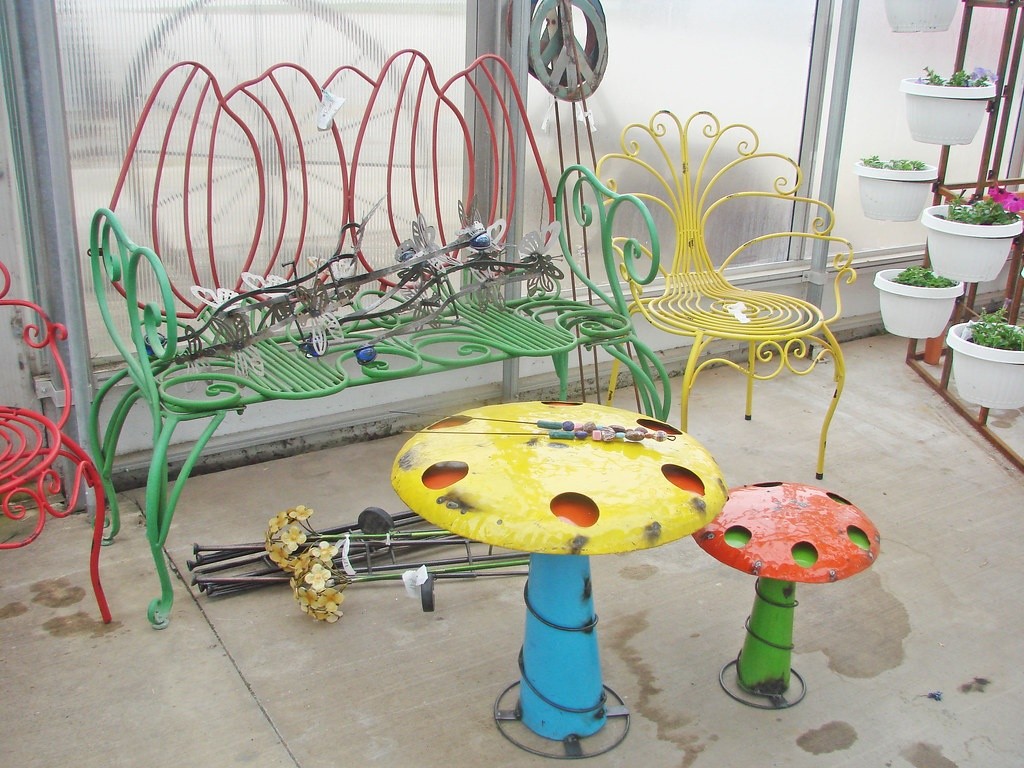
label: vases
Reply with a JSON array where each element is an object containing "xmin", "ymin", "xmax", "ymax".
[
  {"xmin": 900, "ymin": 78, "xmax": 996, "ymax": 144},
  {"xmin": 885, "ymin": 0, "xmax": 960, "ymax": 33},
  {"xmin": 922, "ymin": 205, "xmax": 1023, "ymax": 282},
  {"xmin": 947, "ymin": 322, "xmax": 1024, "ymax": 409}
]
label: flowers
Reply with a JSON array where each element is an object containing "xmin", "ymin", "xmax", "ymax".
[
  {"xmin": 968, "ymin": 297, "xmax": 1024, "ymax": 351},
  {"xmin": 916, "ymin": 64, "xmax": 998, "ymax": 89},
  {"xmin": 948, "ymin": 183, "xmax": 1024, "ymax": 225}
]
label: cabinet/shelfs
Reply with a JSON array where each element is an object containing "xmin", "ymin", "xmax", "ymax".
[{"xmin": 905, "ymin": 0, "xmax": 1024, "ymax": 476}]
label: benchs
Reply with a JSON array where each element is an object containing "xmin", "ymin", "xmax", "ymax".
[{"xmin": 87, "ymin": 50, "xmax": 669, "ymax": 630}]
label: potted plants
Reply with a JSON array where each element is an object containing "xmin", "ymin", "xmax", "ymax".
[
  {"xmin": 854, "ymin": 156, "xmax": 938, "ymax": 222},
  {"xmin": 874, "ymin": 266, "xmax": 965, "ymax": 339}
]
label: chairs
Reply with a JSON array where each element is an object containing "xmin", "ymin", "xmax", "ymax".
[
  {"xmin": 0, "ymin": 260, "xmax": 110, "ymax": 629},
  {"xmin": 590, "ymin": 110, "xmax": 857, "ymax": 479}
]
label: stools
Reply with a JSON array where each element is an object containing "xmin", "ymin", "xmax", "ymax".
[
  {"xmin": 692, "ymin": 481, "xmax": 879, "ymax": 710},
  {"xmin": 388, "ymin": 400, "xmax": 729, "ymax": 760}
]
